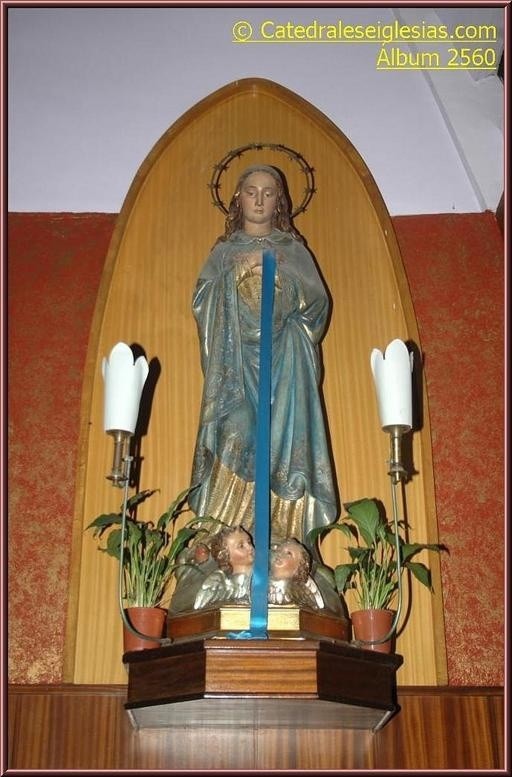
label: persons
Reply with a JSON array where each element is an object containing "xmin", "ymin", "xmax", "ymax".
[
  {"xmin": 268, "ymin": 538, "xmax": 324, "ymax": 612},
  {"xmin": 184, "ymin": 165, "xmax": 341, "ymax": 562},
  {"xmin": 194, "ymin": 526, "xmax": 255, "ymax": 609}
]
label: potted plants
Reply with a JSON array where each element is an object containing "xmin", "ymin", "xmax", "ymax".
[
  {"xmin": 80, "ymin": 482, "xmax": 231, "ymax": 652},
  {"xmin": 302, "ymin": 497, "xmax": 446, "ymax": 655}
]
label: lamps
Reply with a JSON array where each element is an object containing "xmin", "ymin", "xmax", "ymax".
[
  {"xmin": 97, "ymin": 341, "xmax": 175, "ymax": 647},
  {"xmin": 347, "ymin": 337, "xmax": 420, "ymax": 647}
]
